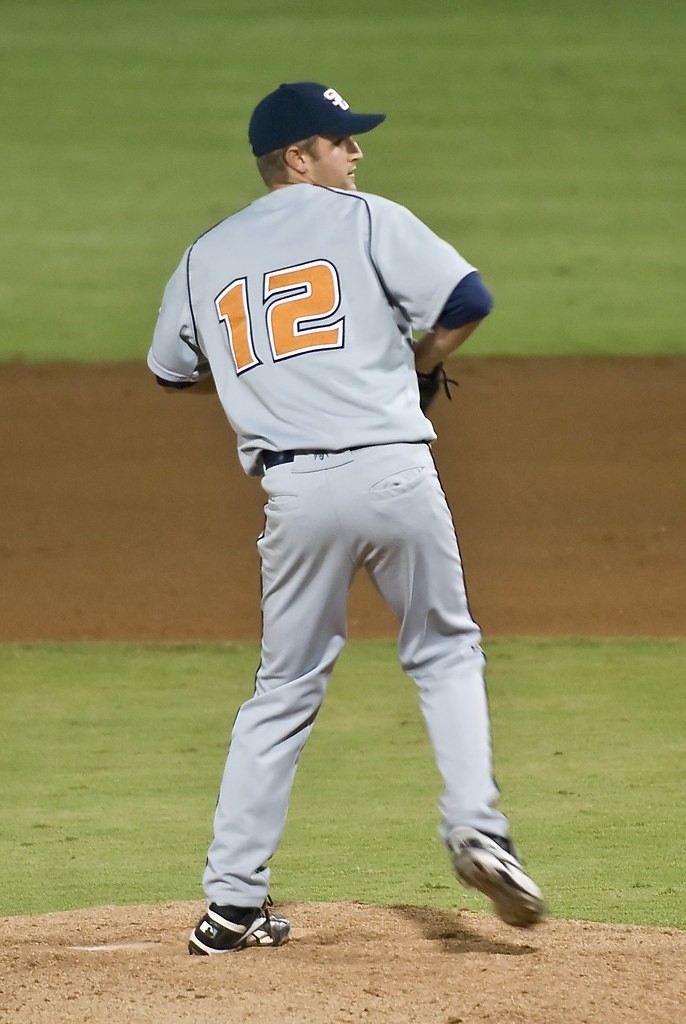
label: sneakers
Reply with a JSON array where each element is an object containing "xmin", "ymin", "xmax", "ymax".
[
  {"xmin": 445, "ymin": 826, "xmax": 544, "ymax": 928},
  {"xmin": 188, "ymin": 894, "xmax": 289, "ymax": 955}
]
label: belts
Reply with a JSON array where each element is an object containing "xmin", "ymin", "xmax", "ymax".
[{"xmin": 264, "ymin": 449, "xmax": 294, "ymax": 470}]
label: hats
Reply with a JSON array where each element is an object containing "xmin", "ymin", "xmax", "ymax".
[{"xmin": 248, "ymin": 84, "xmax": 387, "ymax": 156}]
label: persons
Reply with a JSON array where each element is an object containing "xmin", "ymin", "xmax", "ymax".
[{"xmin": 145, "ymin": 80, "xmax": 549, "ymax": 958}]
